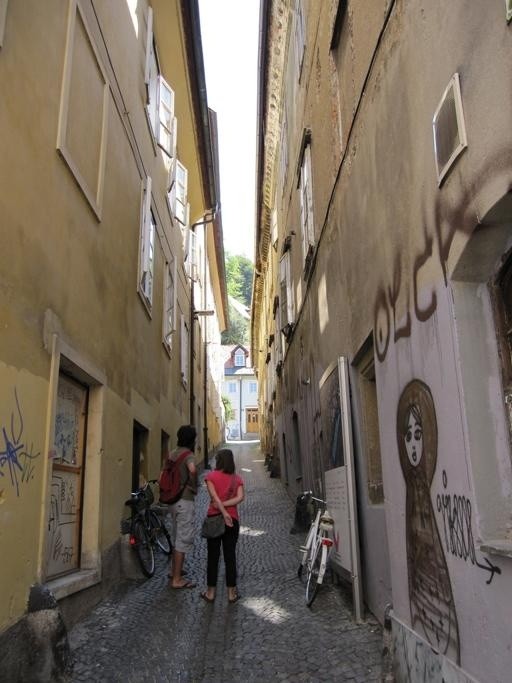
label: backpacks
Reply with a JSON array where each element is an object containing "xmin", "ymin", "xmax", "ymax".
[{"xmin": 158, "ymin": 456, "xmax": 185, "ymax": 504}]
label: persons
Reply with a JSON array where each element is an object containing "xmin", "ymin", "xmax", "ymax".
[
  {"xmin": 159, "ymin": 424, "xmax": 199, "ymax": 589},
  {"xmin": 201, "ymin": 447, "xmax": 244, "ymax": 604},
  {"xmin": 400, "ymin": 403, "xmax": 425, "ymax": 467}
]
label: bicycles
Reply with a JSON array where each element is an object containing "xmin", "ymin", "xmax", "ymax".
[
  {"xmin": 121, "ymin": 476, "xmax": 173, "ymax": 578},
  {"xmin": 296, "ymin": 489, "xmax": 337, "ymax": 608}
]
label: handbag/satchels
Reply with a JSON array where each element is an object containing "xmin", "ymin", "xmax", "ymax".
[
  {"xmin": 290, "ymin": 493, "xmax": 315, "ymax": 533},
  {"xmin": 202, "ymin": 515, "xmax": 225, "ymax": 539}
]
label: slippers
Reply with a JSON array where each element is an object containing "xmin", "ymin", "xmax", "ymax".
[{"xmin": 169, "ymin": 570, "xmax": 241, "ymax": 602}]
